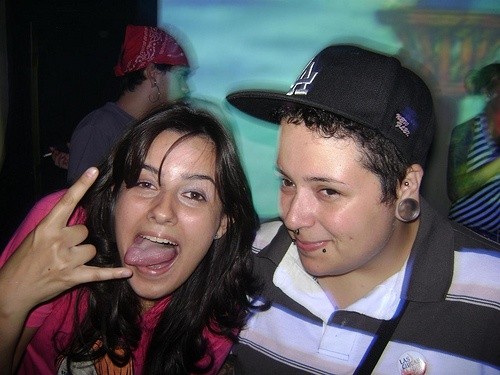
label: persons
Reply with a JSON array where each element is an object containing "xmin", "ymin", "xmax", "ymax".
[
  {"xmin": 63, "ymin": 24, "xmax": 191, "ymax": 187},
  {"xmin": 220, "ymin": 43, "xmax": 500, "ymax": 375},
  {"xmin": 446, "ymin": 62, "xmax": 500, "ymax": 238},
  {"xmin": 49, "ymin": 142, "xmax": 71, "ymax": 170},
  {"xmin": 0, "ymin": 103, "xmax": 273, "ymax": 375}
]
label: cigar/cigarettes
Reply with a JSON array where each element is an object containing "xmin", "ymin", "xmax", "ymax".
[{"xmin": 42, "ymin": 152, "xmax": 52, "ymax": 157}]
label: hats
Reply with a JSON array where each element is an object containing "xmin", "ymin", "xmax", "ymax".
[{"xmin": 225, "ymin": 43, "xmax": 436, "ymax": 166}]
